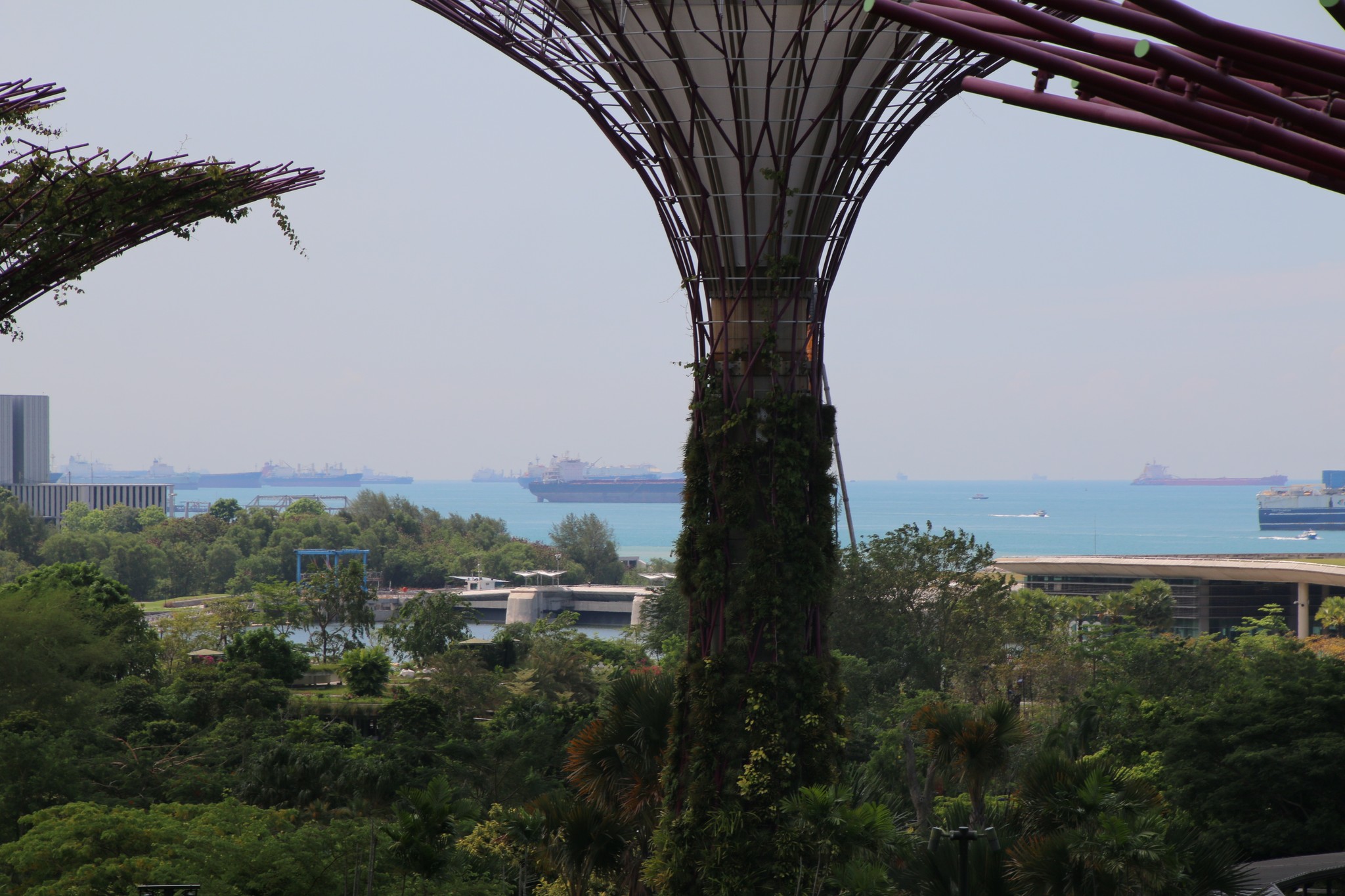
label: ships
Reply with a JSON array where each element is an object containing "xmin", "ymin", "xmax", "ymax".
[
  {"xmin": 1133, "ymin": 460, "xmax": 1289, "ymax": 487},
  {"xmin": 528, "ymin": 454, "xmax": 688, "ymax": 506}
]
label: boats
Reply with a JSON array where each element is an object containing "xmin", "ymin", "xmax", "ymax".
[
  {"xmin": 971, "ymin": 494, "xmax": 989, "ymax": 499},
  {"xmin": 1256, "ymin": 481, "xmax": 1345, "ymax": 532},
  {"xmin": 1033, "ymin": 510, "xmax": 1047, "ymax": 517},
  {"xmin": 1296, "ymin": 528, "xmax": 1317, "ymax": 540},
  {"xmin": 518, "ymin": 460, "xmax": 549, "ymax": 490}
]
